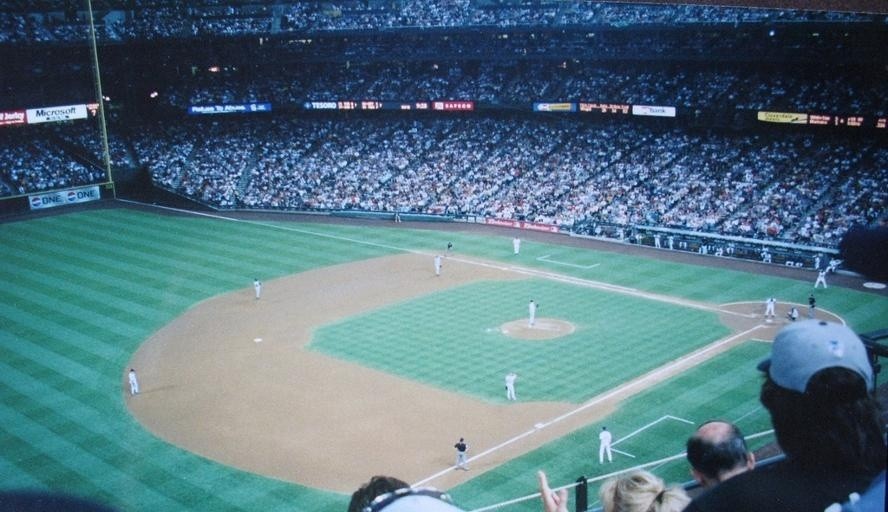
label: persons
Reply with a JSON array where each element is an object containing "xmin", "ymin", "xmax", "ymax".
[
  {"xmin": 765, "ymin": 295, "xmax": 817, "ymax": 320},
  {"xmin": 434, "ymin": 255, "xmax": 441, "ymax": 276},
  {"xmin": 3, "ymin": 2, "xmax": 888, "ymax": 272},
  {"xmin": 529, "ymin": 299, "xmax": 538, "ymax": 325},
  {"xmin": 254, "ymin": 278, "xmax": 261, "ymax": 298},
  {"xmin": 348, "ymin": 476, "xmax": 473, "ymax": 512},
  {"xmin": 539, "ymin": 321, "xmax": 888, "ymax": 512},
  {"xmin": 813, "ymin": 268, "xmax": 828, "ymax": 288},
  {"xmin": 454, "ymin": 437, "xmax": 470, "ymax": 470},
  {"xmin": 505, "ymin": 373, "xmax": 518, "ymax": 399},
  {"xmin": 128, "ymin": 369, "xmax": 139, "ymax": 395}
]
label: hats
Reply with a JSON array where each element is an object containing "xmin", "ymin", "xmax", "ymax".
[{"xmin": 756, "ymin": 319, "xmax": 877, "ymax": 399}]
